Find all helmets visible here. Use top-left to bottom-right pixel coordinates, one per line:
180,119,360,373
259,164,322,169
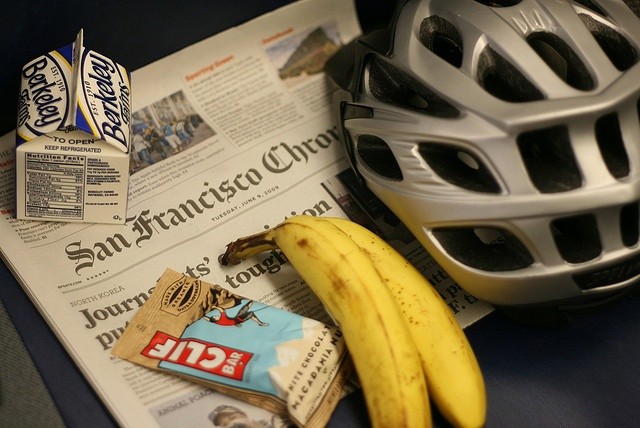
340,0,639,314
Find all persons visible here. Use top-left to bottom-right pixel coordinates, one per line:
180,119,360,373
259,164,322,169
129,113,194,176
208,405,270,428
202,300,269,327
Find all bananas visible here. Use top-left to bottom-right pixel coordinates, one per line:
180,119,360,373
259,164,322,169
223,215,488,428
